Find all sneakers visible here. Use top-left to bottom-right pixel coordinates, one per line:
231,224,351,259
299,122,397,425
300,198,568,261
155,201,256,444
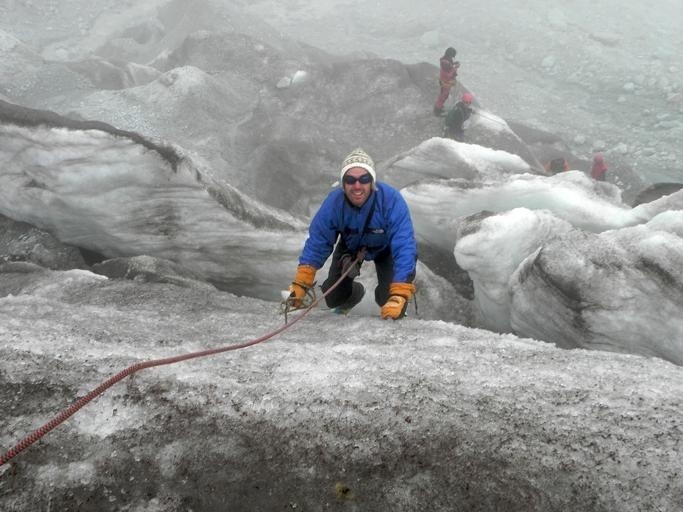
334,282,364,315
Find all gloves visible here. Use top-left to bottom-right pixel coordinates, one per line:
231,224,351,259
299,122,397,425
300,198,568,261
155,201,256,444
289,264,315,308
382,283,415,320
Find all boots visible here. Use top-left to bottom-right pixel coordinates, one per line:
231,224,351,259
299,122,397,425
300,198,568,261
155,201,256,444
434,107,446,117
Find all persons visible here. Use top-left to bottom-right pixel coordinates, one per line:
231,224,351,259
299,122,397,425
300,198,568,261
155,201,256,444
545,159,569,176
434,48,460,118
289,149,419,321
444,92,476,141
593,154,608,179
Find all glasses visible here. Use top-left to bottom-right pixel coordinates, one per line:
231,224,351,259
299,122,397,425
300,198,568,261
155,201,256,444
343,174,371,184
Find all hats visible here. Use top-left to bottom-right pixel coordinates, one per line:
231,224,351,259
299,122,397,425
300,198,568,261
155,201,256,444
445,47,456,57
341,147,376,191
462,94,471,103
